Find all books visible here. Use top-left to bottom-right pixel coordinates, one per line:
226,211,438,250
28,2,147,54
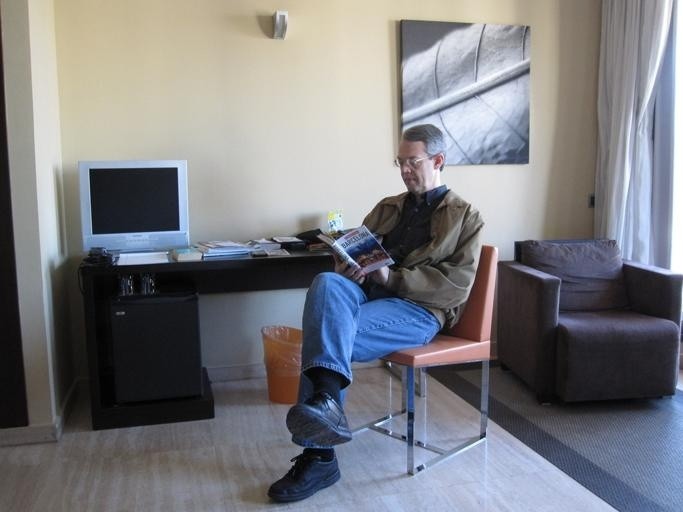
172,238,281,263
316,225,395,278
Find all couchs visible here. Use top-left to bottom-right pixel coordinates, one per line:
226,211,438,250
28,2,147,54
497,238,682,405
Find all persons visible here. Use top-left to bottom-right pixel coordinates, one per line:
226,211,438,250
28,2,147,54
265,124,485,503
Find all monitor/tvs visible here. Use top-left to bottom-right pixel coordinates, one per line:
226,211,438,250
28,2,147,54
79,160,189,252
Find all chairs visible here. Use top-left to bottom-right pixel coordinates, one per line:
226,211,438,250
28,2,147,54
349,238,499,475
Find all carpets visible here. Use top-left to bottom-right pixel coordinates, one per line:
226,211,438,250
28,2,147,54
423,358,681,510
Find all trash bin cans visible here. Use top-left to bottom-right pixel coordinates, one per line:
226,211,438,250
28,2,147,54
261,325,304,404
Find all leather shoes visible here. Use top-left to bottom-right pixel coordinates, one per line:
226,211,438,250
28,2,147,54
286,392,352,445
266,449,341,502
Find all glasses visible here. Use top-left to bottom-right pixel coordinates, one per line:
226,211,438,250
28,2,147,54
394,155,434,169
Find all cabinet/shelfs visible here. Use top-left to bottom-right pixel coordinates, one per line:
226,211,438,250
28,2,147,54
78,239,350,430
